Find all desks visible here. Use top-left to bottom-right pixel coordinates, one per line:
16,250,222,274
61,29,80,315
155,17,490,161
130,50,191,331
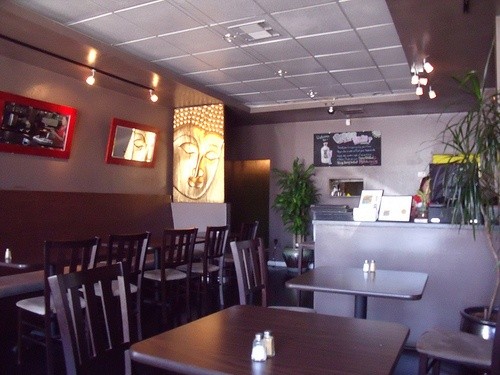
0,241,204,298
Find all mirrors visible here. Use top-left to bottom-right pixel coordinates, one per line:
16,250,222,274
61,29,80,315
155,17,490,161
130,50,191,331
104,118,161,169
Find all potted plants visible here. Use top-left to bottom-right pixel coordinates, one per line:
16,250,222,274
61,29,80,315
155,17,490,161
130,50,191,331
417,191,430,219
434,70,500,340
270,157,322,276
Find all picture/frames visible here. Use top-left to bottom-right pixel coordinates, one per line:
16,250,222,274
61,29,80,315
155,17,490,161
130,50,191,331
1,90,78,159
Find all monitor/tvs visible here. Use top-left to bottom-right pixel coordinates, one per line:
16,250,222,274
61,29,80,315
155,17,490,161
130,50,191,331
429,163,478,200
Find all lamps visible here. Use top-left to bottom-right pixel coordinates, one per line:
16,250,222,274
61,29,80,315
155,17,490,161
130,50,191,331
149,89,158,103
86,70,96,86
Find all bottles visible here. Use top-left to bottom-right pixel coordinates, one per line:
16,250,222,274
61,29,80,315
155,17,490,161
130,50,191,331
363,272,368,280
251,362,265,375
370,260,376,272
321,143,330,164
363,260,369,272
5,248,11,258
5,259,11,263
370,272,375,280
251,333,266,361
263,330,275,357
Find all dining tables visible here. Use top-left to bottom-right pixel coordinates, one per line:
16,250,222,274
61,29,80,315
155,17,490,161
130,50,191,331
286,263,429,319
124,304,411,375
1,261,33,273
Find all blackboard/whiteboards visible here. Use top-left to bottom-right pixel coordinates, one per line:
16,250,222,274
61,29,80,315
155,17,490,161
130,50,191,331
312,131,382,167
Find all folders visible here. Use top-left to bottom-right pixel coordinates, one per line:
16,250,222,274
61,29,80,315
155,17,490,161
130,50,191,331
310,203,354,221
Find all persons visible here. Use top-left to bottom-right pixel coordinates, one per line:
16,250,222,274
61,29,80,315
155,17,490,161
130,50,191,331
412,176,429,217
1,102,68,151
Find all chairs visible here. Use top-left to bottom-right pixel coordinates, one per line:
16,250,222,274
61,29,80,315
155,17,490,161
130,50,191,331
16,237,102,365
144,221,259,331
230,237,268,306
95,231,151,347
47,259,135,375
416,284,500,374
